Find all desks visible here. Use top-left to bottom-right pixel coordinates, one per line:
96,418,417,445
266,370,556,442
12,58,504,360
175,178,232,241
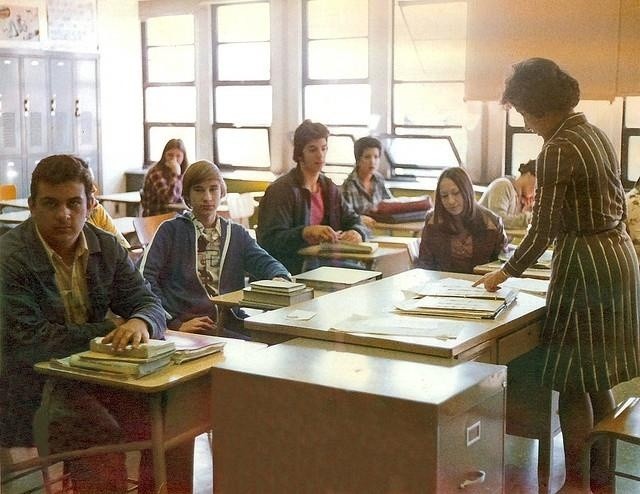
0,198,28,214
31,330,268,494
473,259,552,280
298,242,413,280
372,221,425,239
244,268,562,494
163,202,251,229
209,276,338,346
111,216,142,250
0,210,31,223
504,229,527,239
96,190,143,204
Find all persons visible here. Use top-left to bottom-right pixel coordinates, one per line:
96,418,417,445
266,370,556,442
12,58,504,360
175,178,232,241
0,154,194,494
139,139,190,217
477,158,537,231
625,177,640,262
137,161,294,341
256,120,369,274
78,157,133,253
472,58,640,494
417,168,508,273
340,136,397,229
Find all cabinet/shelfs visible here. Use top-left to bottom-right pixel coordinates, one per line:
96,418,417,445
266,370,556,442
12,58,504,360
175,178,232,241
0,48,104,212
211,337,508,494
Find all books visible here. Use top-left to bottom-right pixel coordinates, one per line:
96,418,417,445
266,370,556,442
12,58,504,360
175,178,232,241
319,239,379,254
239,279,314,308
296,265,382,292
390,277,519,319
498,243,554,269
50,328,226,380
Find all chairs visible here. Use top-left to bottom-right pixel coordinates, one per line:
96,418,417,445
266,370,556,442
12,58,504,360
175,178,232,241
581,396,640,494
0,183,18,204
133,212,180,250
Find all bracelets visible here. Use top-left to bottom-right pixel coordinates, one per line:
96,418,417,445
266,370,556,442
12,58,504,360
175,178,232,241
501,268,509,279
